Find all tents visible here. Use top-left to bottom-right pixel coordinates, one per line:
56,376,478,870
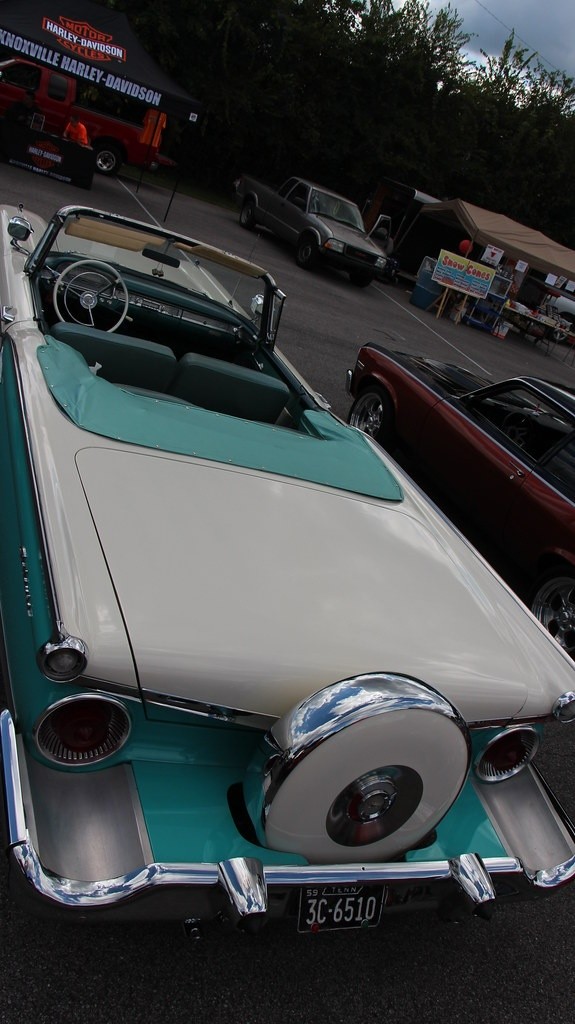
0,0,207,221
393,198,575,331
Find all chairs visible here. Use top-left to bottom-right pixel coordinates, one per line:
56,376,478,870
54,324,178,392
172,350,291,425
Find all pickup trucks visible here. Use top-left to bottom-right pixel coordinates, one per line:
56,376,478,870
237,173,392,289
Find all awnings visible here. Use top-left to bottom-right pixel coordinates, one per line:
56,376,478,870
386,178,444,244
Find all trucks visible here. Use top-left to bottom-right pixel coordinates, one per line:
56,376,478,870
0,58,178,178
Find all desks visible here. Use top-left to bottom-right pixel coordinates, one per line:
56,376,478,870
491,303,575,369
0,119,94,191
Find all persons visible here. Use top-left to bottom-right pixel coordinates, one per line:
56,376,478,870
64,114,89,146
5,90,43,130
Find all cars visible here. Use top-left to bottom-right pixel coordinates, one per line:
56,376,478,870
1,202,575,932
347,339,575,664
540,293,575,345
